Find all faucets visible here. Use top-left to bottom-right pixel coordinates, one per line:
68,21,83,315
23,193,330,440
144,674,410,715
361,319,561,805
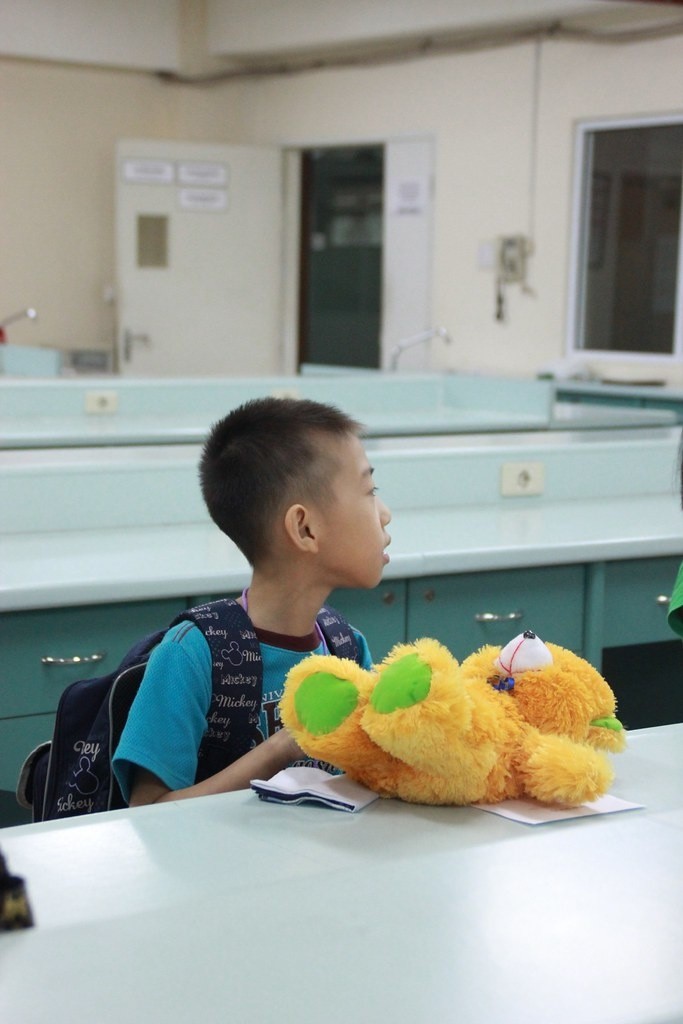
0,307,39,327
379,326,452,373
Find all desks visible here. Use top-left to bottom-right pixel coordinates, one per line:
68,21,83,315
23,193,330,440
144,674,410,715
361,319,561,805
0,441,683,612
0,374,558,453
0,722,682,1024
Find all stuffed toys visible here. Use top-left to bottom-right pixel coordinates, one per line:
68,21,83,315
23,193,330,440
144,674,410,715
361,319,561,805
276,628,628,811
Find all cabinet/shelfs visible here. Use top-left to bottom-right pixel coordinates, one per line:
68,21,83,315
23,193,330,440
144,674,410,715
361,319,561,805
214,561,588,762
588,550,683,675
0,598,190,828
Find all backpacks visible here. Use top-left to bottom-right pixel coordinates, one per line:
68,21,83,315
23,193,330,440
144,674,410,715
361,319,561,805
17,599,360,822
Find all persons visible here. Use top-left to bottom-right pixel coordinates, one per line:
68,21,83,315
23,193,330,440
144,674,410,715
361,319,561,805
110,396,394,810
